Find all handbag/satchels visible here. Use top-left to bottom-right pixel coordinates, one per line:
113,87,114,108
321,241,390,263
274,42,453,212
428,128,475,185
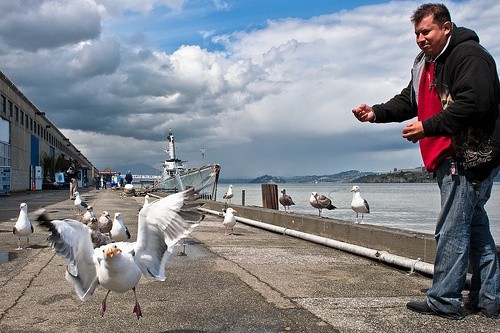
456,131,500,181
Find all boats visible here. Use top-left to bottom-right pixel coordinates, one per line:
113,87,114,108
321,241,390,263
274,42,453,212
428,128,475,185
132,127,221,202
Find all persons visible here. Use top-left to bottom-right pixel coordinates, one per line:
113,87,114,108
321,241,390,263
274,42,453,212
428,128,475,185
67,161,80,199
352,3,500,319
102,174,107,189
117,171,124,189
126,170,133,184
95,173,100,189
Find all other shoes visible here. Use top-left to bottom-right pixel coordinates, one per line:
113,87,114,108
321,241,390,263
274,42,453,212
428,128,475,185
464,301,500,319
407,300,436,314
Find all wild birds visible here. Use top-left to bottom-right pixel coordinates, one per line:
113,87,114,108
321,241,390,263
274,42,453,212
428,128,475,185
223,183,233,203
112,211,131,242
287,211,287,212
351,185,369,224
74,191,87,213
310,191,337,216
279,188,295,212
82,205,94,224
13,202,34,249
87,216,99,231
98,210,112,235
223,207,237,230
36,187,204,320
